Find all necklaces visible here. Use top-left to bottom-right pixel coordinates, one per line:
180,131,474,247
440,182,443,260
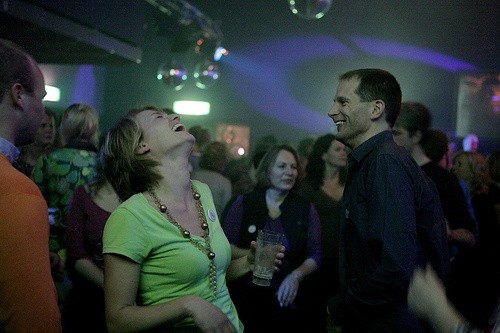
148,180,218,298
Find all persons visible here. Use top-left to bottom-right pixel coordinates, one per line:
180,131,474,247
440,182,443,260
100,105,244,333
326,69,446,333
408,264,500,333
0,43,63,333
223,144,321,333
186,102,500,246
18,103,130,333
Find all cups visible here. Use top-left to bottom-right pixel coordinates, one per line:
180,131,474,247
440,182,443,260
252,230,282,288
47,207,61,227
